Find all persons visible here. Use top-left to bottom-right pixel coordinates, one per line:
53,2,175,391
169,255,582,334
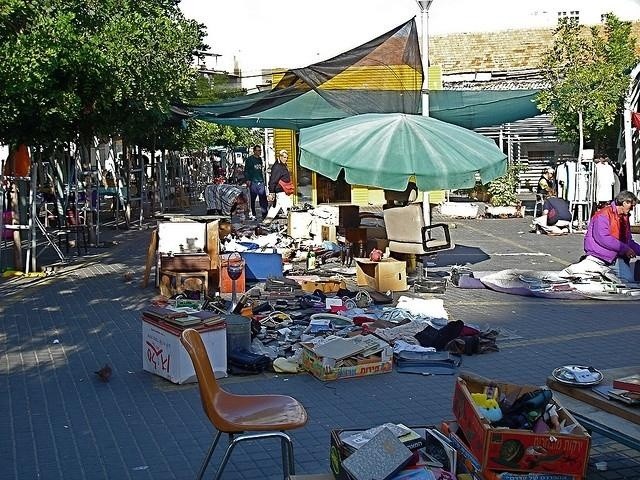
578,190,640,286
243,144,268,219
202,184,248,224
537,166,556,220
538,192,572,228
261,148,295,224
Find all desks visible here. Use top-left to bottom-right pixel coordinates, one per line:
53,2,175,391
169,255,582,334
220,247,283,294
545,358,640,454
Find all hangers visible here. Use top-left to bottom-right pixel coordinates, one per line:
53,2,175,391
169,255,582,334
597,152,611,165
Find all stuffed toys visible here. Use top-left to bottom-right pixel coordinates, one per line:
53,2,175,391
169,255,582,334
471,389,505,422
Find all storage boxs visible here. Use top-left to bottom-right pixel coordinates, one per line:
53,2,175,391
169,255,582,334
139,303,231,385
284,204,409,293
329,420,569,480
452,373,592,480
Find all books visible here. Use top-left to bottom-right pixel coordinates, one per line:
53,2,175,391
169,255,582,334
309,332,385,364
332,420,472,480
139,304,227,332
589,371,640,406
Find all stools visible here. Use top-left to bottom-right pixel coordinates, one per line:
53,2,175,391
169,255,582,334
156,267,210,297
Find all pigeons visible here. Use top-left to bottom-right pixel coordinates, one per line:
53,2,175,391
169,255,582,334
94,362,115,384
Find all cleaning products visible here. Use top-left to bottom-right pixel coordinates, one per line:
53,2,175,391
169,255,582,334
359,240,366,256
340,247,345,265
346,243,353,266
307,244,316,270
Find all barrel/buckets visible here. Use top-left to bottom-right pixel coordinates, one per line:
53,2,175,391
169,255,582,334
224,314,252,355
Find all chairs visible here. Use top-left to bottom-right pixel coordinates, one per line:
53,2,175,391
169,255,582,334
380,200,456,289
532,191,549,219
179,326,310,479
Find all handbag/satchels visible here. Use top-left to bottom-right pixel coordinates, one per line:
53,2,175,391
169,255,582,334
278,176,295,195
546,208,559,225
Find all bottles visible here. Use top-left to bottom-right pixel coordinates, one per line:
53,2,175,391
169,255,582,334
308,248,316,270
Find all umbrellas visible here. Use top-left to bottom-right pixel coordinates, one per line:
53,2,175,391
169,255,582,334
297,111,509,194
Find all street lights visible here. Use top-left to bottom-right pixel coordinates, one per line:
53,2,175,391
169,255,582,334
415,0,433,233
